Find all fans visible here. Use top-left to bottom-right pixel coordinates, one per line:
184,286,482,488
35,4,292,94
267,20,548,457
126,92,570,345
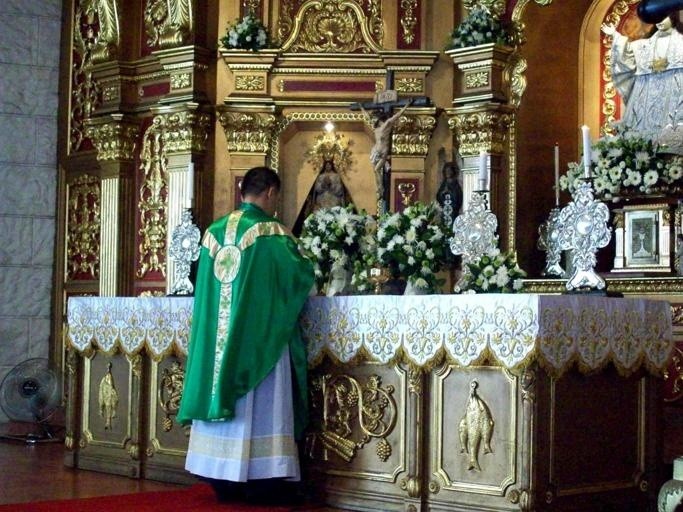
1,357,66,445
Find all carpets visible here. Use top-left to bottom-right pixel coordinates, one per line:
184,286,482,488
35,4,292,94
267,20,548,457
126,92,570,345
0,483,329,512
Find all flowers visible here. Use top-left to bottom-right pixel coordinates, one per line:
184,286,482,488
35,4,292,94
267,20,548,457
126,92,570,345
446,6,515,47
376,201,453,287
291,207,377,293
559,122,683,196
453,236,526,293
219,14,280,49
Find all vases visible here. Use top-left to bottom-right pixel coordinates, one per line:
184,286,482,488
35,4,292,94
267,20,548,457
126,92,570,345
403,277,433,293
329,269,355,295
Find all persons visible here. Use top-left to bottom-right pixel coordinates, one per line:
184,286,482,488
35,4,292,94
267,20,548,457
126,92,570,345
292,141,358,238
357,96,413,200
437,162,462,219
599,7,682,140
173,165,316,507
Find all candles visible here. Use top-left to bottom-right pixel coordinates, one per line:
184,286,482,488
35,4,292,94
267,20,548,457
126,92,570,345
554,142,560,197
188,163,194,200
582,125,590,178
480,150,487,181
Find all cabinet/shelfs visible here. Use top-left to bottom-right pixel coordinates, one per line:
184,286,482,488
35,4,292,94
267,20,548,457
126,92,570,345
63,294,663,512
